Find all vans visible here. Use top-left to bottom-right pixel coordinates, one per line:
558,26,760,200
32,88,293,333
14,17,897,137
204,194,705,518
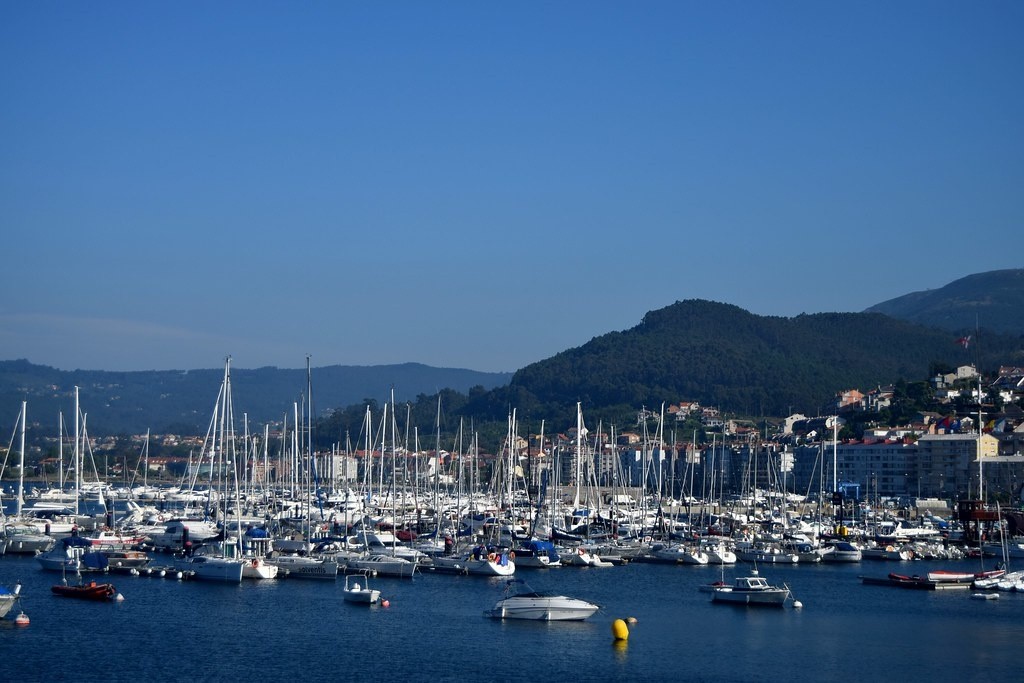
614,494,636,504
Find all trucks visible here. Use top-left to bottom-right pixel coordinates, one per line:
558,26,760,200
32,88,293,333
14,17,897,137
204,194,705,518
0,353,1024,589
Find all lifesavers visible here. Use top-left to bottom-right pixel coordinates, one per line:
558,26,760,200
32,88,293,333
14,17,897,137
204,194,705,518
645,536,651,541
665,520,670,525
510,552,515,558
322,524,328,531
578,548,585,556
252,560,259,568
158,516,163,521
488,553,495,560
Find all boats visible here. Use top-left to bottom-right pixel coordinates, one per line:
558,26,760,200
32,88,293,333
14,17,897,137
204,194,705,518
713,576,790,607
973,593,999,600
0,587,21,619
484,578,600,621
344,574,380,602
51,573,117,599
